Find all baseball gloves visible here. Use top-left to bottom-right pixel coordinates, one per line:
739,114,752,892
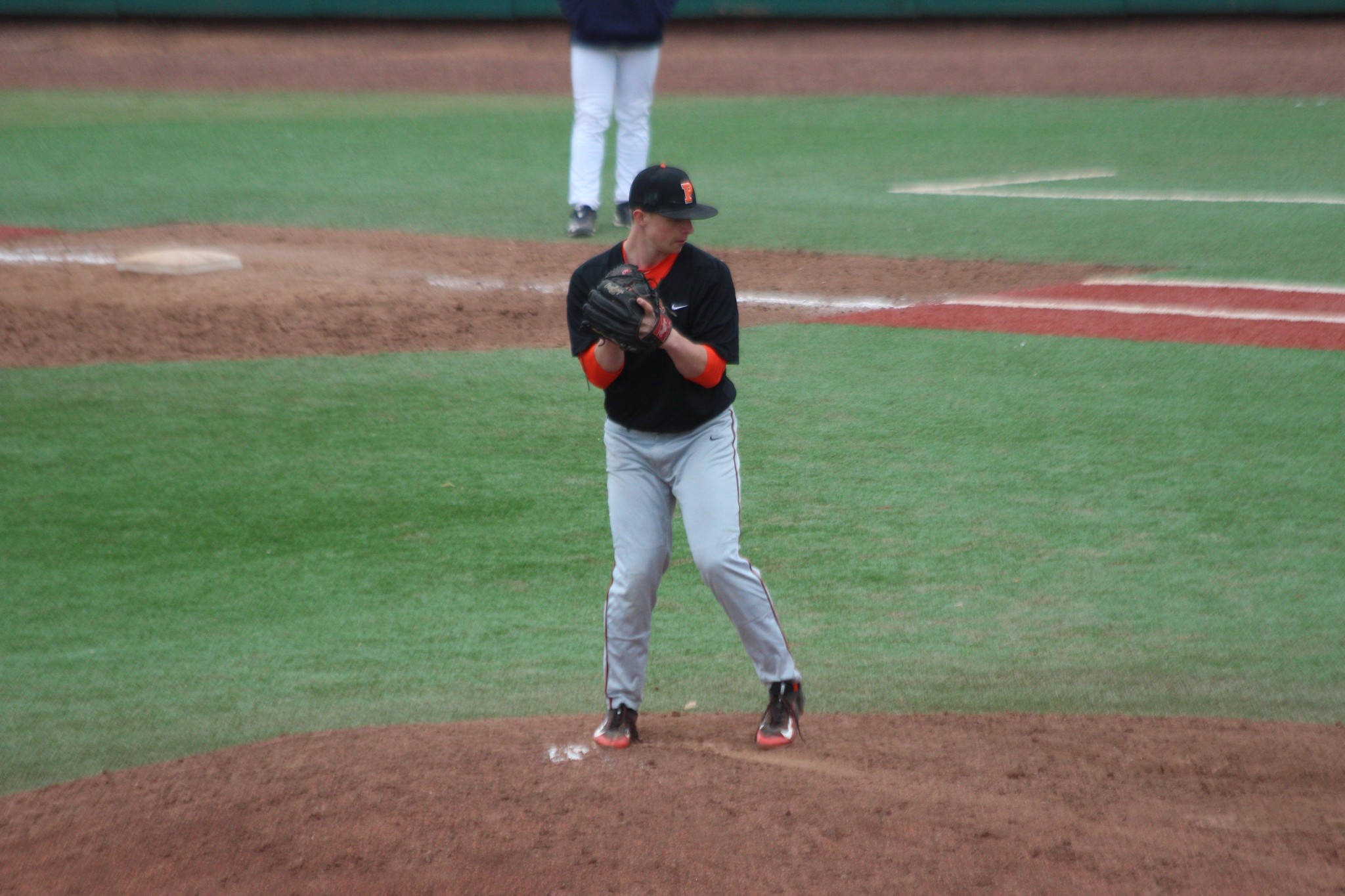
582,264,674,352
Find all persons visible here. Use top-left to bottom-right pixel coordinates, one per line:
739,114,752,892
554,0,676,239
566,163,806,747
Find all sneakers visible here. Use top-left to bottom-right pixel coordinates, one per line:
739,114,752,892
612,201,633,227
594,702,639,750
755,679,808,750
566,205,597,237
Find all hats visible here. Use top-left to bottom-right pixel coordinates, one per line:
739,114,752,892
629,163,719,220
